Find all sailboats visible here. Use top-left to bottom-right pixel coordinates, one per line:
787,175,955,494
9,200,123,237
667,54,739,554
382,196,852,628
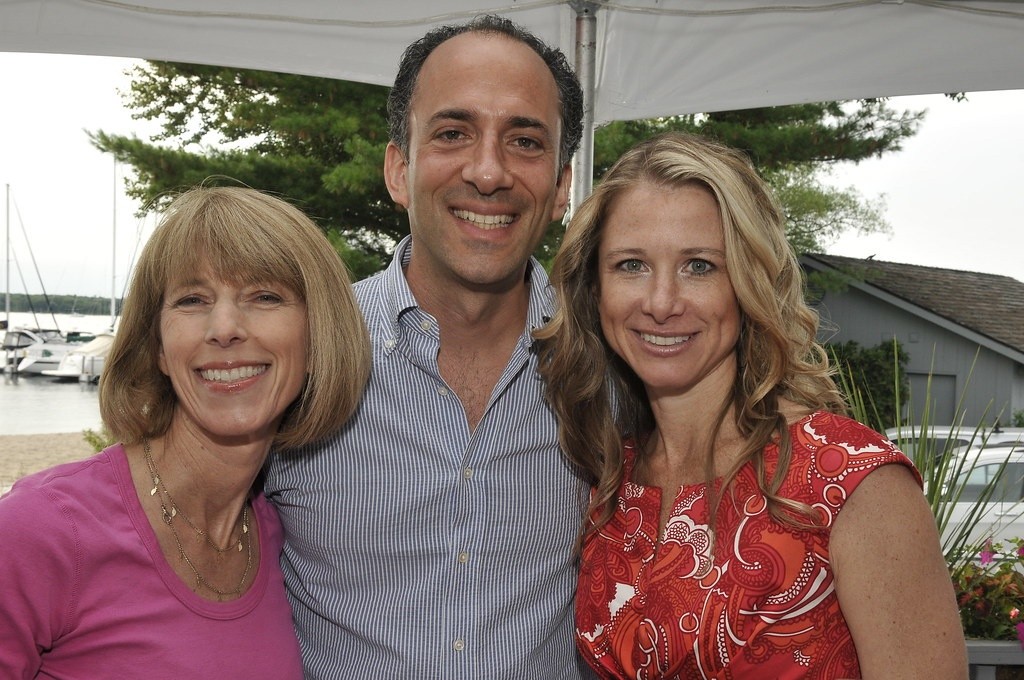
0,140,164,383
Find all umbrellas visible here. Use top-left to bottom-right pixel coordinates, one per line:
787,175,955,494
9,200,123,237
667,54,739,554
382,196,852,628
0,0,1024,224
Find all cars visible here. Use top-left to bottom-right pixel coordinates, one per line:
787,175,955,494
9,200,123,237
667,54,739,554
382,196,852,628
884,424,1024,585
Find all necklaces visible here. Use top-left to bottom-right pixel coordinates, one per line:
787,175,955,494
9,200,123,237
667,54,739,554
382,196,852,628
141,440,253,603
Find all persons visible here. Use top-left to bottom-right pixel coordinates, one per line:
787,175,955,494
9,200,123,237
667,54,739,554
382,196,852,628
532,131,967,680
263,18,615,680
0,185,370,680
